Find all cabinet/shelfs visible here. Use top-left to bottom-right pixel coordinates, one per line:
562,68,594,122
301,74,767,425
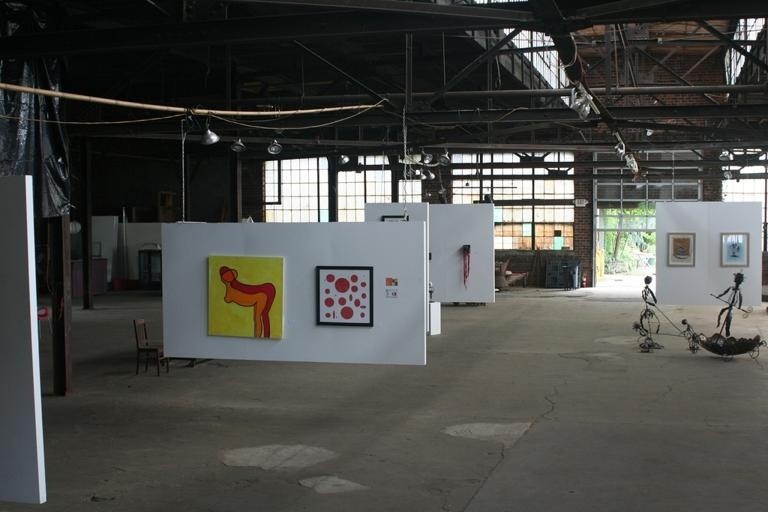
70,257,107,299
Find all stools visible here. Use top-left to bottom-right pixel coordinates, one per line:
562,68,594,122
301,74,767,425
38,307,52,339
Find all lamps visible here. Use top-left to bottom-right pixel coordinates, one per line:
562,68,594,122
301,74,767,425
417,148,450,181
199,119,283,155
338,153,350,165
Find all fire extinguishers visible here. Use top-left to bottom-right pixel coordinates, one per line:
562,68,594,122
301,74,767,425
581,271,587,287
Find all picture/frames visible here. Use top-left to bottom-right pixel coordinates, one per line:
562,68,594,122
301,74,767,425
315,265,375,328
666,233,696,268
720,232,750,267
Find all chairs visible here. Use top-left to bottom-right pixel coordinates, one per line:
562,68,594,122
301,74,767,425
133,317,169,377
